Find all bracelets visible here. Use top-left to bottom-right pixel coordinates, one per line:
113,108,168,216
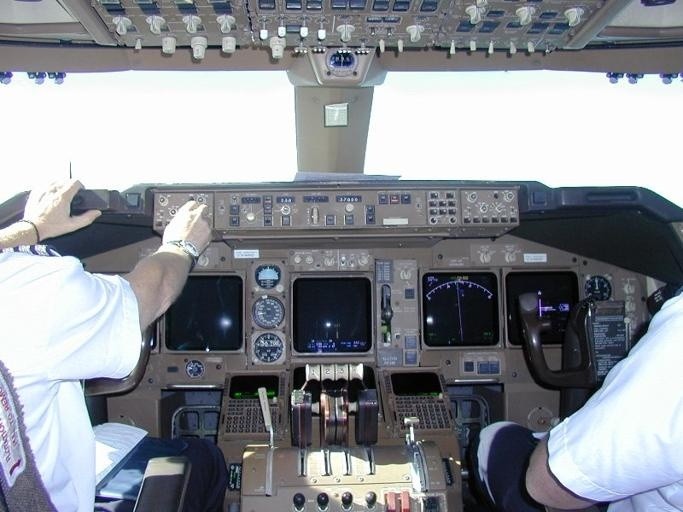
19,218,40,244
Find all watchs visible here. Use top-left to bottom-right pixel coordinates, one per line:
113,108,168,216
165,239,200,273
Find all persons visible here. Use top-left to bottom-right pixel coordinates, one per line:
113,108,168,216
0,176,230,512
467,289,683,512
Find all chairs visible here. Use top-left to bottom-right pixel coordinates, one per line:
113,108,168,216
0,359,192,512
